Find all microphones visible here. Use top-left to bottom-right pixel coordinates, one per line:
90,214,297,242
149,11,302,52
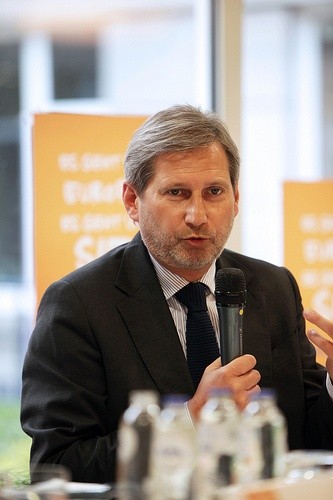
215,268,247,368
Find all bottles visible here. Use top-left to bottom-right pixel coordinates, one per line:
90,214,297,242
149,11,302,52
114,389,161,500
147,393,194,500
191,387,247,500
243,387,288,480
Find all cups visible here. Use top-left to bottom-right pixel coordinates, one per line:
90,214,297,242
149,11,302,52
0,466,70,500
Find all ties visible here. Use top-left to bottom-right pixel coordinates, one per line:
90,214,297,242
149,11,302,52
172,282,220,394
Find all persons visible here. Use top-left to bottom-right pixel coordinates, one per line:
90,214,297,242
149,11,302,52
21,104,333,481
